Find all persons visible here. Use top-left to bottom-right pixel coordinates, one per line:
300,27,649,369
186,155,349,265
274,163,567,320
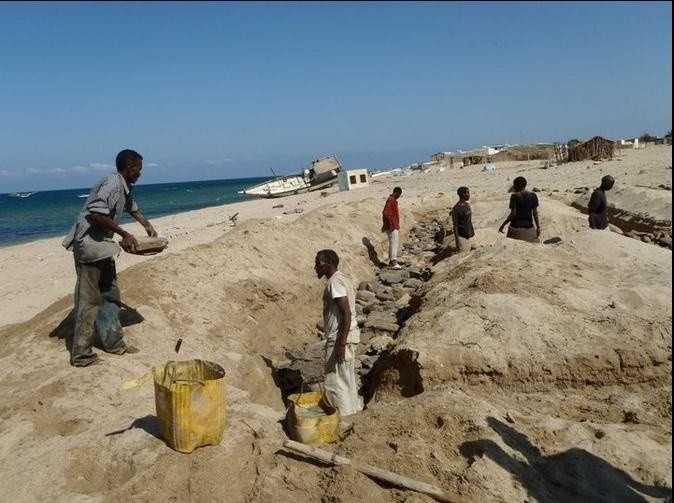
59,149,156,365
313,249,364,417
450,186,478,253
588,174,615,232
381,186,402,269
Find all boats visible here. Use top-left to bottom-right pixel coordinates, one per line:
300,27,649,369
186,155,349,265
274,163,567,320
8,190,40,198
237,153,342,198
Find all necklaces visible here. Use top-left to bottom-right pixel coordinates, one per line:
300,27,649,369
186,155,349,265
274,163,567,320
499,176,541,243
